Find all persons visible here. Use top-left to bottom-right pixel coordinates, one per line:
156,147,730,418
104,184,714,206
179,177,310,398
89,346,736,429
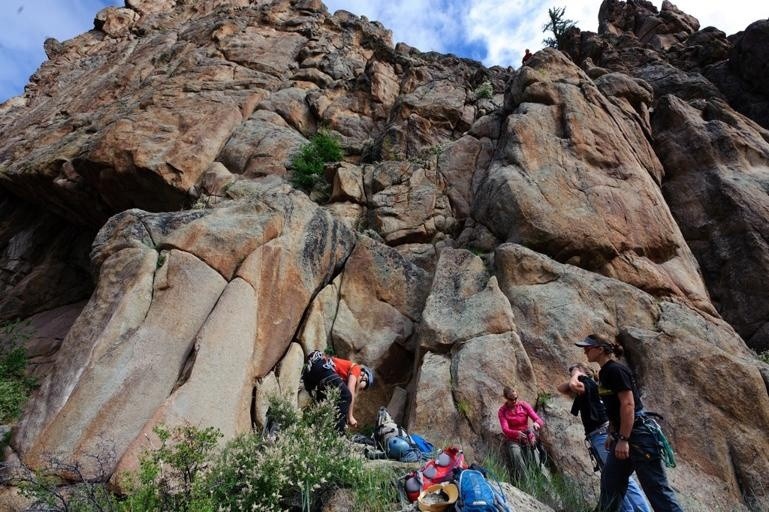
574,332,686,511
302,349,374,435
498,385,565,511
558,360,652,511
522,48,533,65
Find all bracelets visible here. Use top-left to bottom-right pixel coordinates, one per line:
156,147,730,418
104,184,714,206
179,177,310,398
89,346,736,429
617,432,629,441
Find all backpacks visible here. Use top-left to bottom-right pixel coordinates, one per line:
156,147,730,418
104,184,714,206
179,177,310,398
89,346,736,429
453,463,511,512
397,447,468,506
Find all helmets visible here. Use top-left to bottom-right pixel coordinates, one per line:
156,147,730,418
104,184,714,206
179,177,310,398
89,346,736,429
360,367,374,391
387,438,410,458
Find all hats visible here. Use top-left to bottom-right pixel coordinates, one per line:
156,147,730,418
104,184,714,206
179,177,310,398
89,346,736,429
415,482,459,512
574,336,601,349
